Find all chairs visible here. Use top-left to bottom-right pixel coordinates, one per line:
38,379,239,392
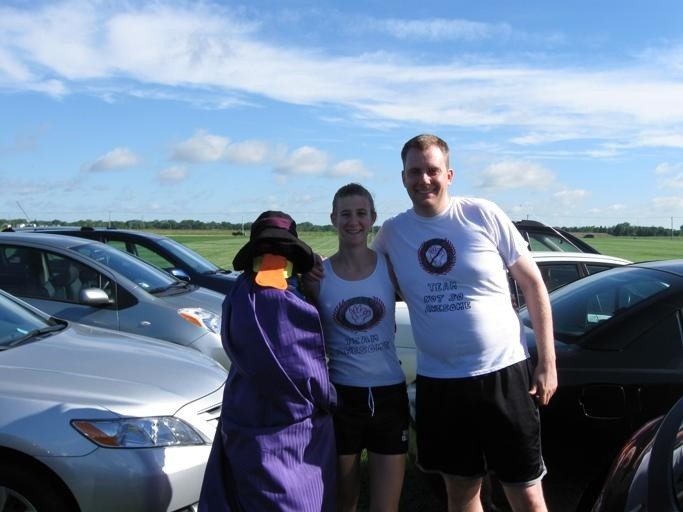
48,254,98,303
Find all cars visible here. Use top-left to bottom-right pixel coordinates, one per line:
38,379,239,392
1,289,231,511
1,231,232,379
412,259,682,479
7,227,243,297
391,251,645,400
517,219,599,251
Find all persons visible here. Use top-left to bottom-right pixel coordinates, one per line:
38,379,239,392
192,210,342,512
297,181,521,511
293,131,560,511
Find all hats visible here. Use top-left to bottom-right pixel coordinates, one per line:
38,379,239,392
232,211,315,273
